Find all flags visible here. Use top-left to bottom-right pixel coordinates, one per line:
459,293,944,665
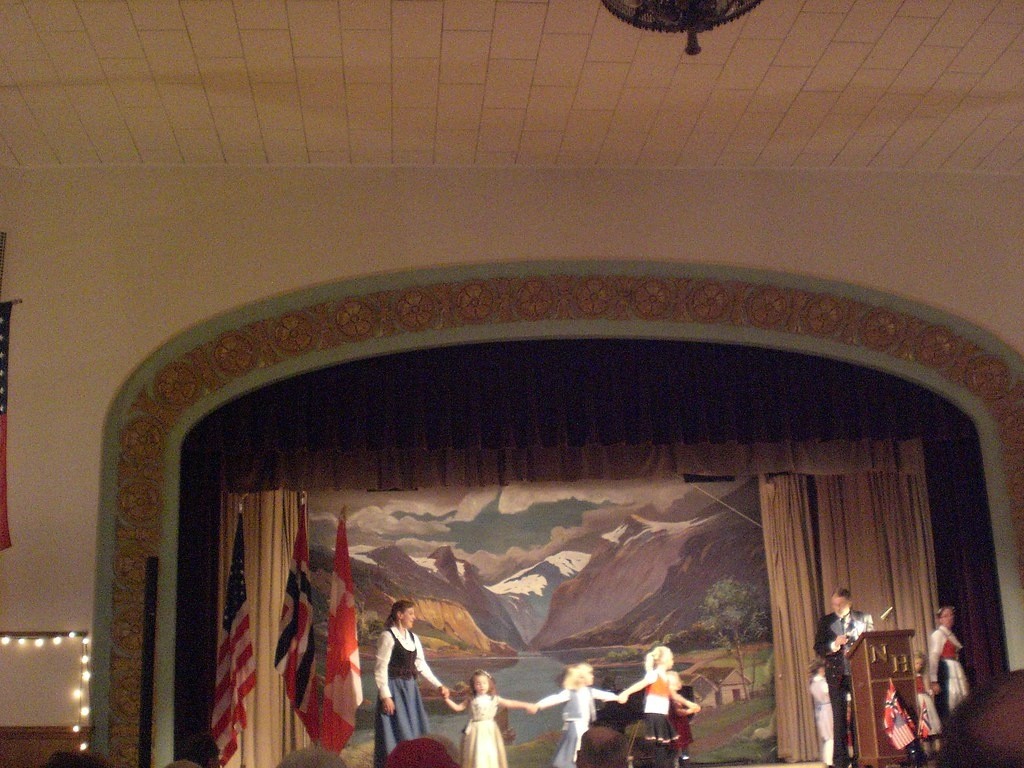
884,678,932,750
212,490,363,768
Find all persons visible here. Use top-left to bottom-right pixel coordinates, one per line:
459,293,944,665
374,600,701,768
937,667,1024,768
914,653,943,760
164,760,203,768
44,752,114,768
276,747,348,768
928,605,971,721
810,587,873,768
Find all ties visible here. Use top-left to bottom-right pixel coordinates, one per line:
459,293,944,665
840,618,847,635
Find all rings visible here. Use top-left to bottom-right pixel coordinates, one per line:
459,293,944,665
384,707,387,709
